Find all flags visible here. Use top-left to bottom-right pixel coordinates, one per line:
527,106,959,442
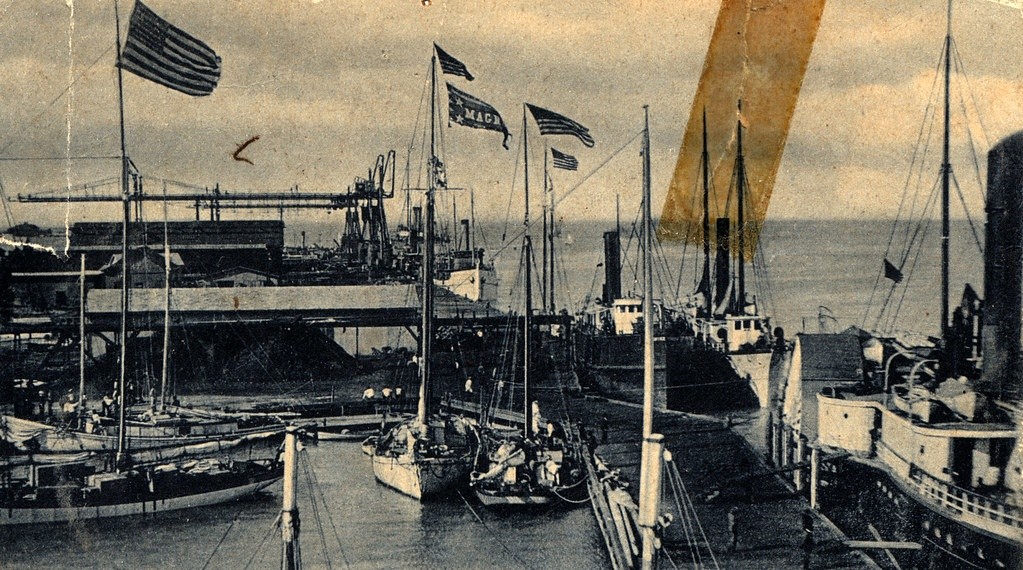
446,85,512,150
885,259,903,284
435,42,475,82
116,0,221,97
550,147,579,171
526,103,595,148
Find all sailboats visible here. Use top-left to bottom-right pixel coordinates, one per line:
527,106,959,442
577,96,770,411
819,2,1019,569
371,42,482,500
0,0,301,521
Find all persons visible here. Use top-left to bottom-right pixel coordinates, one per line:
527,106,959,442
40,376,181,438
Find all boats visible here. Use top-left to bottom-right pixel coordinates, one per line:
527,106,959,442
480,100,587,511
